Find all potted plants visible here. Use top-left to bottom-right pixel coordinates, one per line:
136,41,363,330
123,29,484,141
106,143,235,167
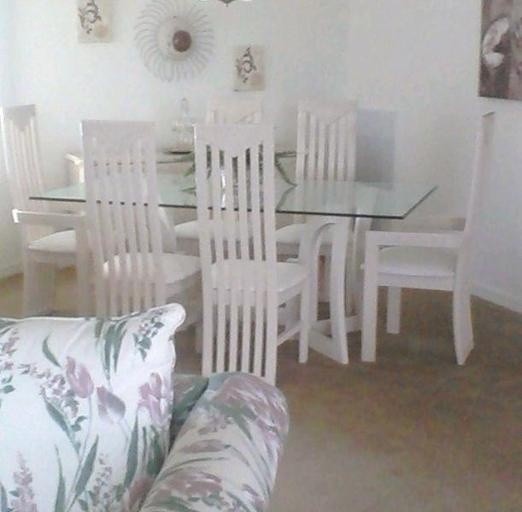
156,112,300,193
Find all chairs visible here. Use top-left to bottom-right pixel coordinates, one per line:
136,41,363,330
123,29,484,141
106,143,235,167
192,121,311,388
172,91,270,258
275,100,362,314
357,111,498,365
80,118,203,353
0,102,89,315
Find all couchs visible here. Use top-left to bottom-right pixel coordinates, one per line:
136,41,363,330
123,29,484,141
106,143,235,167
140,370,291,512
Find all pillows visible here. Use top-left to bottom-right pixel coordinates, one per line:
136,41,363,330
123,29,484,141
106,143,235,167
1,301,187,511
